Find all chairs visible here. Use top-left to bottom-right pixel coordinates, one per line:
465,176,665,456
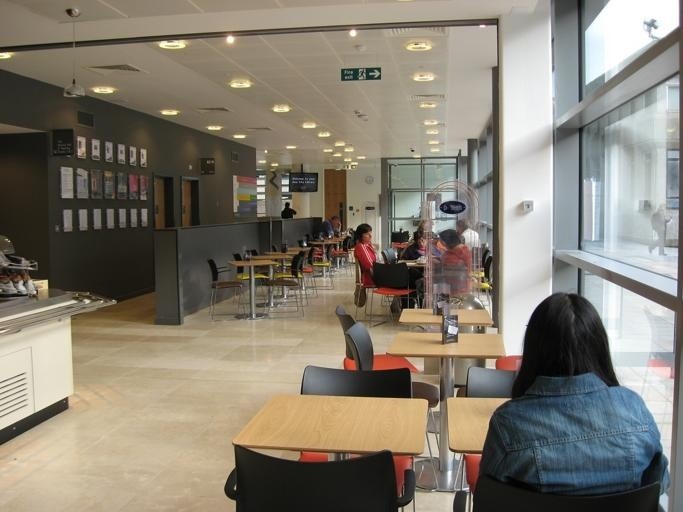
452,453,668,511
295,364,415,512
495,357,521,372
345,322,443,493
223,439,416,511
355,228,494,327
454,366,522,511
335,307,421,374
206,228,357,322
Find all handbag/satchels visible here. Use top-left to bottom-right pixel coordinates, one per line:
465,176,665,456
354,285,366,306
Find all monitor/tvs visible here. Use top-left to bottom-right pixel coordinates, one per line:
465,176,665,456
289,172,318,192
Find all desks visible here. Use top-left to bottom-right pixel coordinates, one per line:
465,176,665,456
387,331,505,492
445,395,514,455
399,307,495,433
232,391,430,465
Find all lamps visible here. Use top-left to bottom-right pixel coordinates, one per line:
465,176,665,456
63,9,85,97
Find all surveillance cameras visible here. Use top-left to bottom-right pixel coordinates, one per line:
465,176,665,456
409,145,417,153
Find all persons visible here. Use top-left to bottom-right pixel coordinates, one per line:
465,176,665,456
647,202,673,257
355,218,481,315
281,202,296,218
479,292,669,496
315,216,342,263
